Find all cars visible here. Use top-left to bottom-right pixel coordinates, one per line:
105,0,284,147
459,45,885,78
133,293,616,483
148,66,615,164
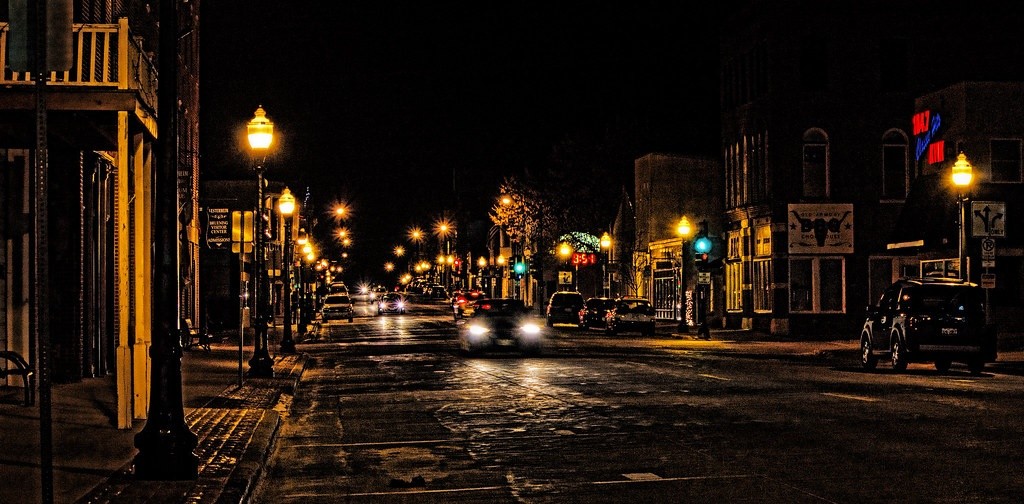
450,290,542,353
606,296,656,337
322,295,354,322
377,293,406,315
358,284,388,303
394,279,449,301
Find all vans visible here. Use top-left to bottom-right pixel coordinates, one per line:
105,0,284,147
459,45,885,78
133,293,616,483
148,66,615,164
329,283,348,295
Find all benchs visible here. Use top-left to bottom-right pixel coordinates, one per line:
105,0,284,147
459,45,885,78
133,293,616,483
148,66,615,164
178,318,212,352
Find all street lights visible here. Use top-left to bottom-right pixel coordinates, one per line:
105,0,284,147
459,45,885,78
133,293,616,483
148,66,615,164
950,155,974,276
601,233,611,297
561,241,569,291
297,233,322,333
278,187,299,355
248,104,276,376
499,254,505,299
480,257,486,291
678,216,692,331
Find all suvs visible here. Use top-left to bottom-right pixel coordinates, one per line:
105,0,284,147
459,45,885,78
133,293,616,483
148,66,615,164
860,278,997,374
544,290,585,327
579,298,616,332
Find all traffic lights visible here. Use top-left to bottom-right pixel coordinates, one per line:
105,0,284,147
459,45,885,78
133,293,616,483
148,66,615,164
702,254,709,271
454,260,459,271
